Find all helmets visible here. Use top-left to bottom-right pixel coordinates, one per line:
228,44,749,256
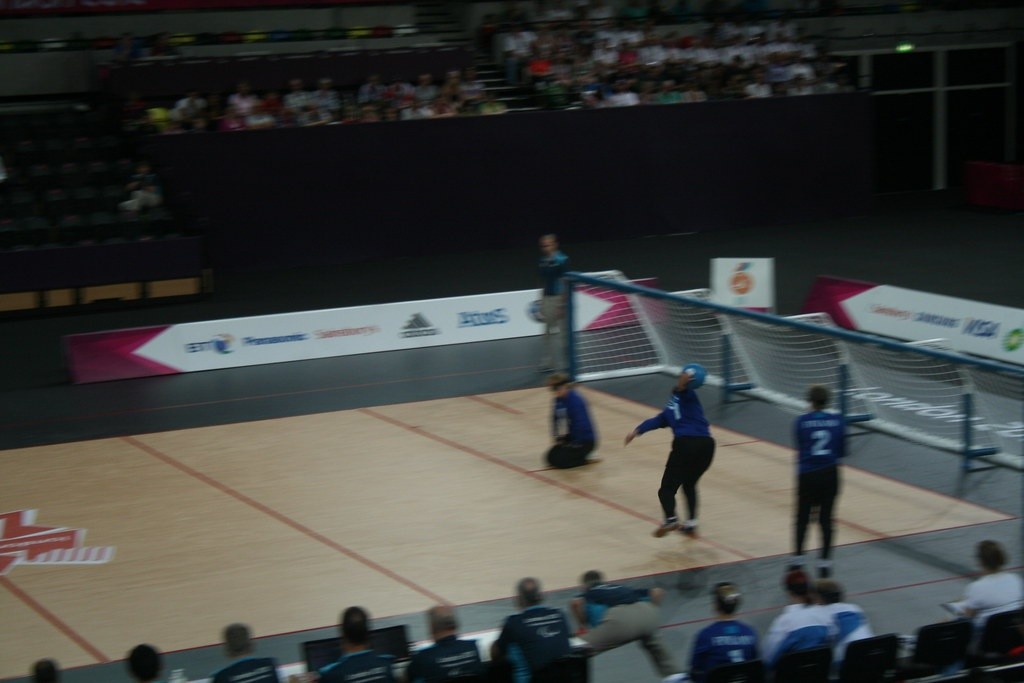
682,363,706,391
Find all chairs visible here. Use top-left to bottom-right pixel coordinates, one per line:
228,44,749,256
914,620,973,667
974,661,1024,683
977,610,1024,654
703,658,765,683
0,126,181,250
529,653,589,683
839,634,899,683
771,647,832,683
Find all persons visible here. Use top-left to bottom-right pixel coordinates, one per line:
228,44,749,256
307,606,402,683
402,604,486,683
945,539,1023,639
625,362,715,538
758,566,841,664
542,372,595,469
566,569,689,683
812,581,876,683
31,658,62,683
488,576,572,682
783,384,850,577
126,643,164,683
71,0,873,232
537,236,573,375
685,582,760,683
209,623,284,683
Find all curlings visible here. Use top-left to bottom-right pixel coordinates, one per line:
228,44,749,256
679,363,708,392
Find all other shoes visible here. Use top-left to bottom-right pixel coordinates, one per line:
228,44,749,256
789,555,805,571
680,518,699,533
817,558,831,579
653,518,679,537
